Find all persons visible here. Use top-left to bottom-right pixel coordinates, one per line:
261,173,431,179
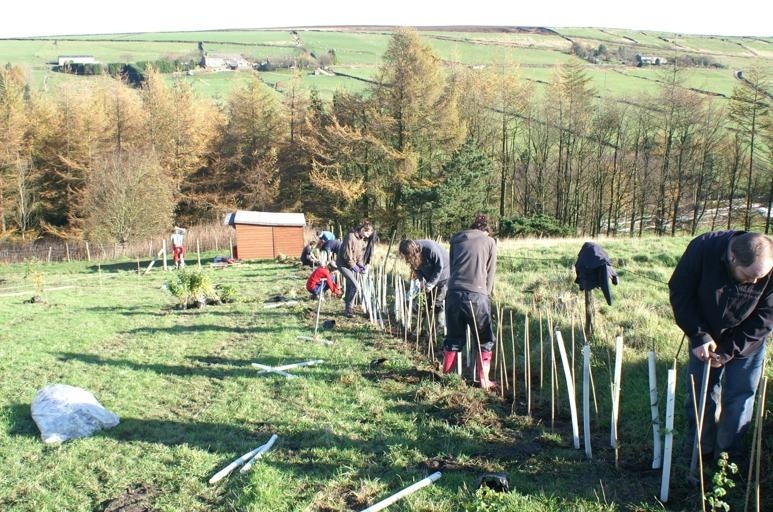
441,214,497,391
306,260,343,303
667,229,773,477
318,238,342,261
299,240,320,267
335,219,375,318
316,230,336,241
170,224,187,269
398,238,451,340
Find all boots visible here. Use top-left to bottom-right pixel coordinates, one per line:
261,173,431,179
476,350,497,390
343,299,356,318
442,349,457,375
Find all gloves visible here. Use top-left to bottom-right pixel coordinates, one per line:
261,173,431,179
356,263,366,273
351,265,359,272
